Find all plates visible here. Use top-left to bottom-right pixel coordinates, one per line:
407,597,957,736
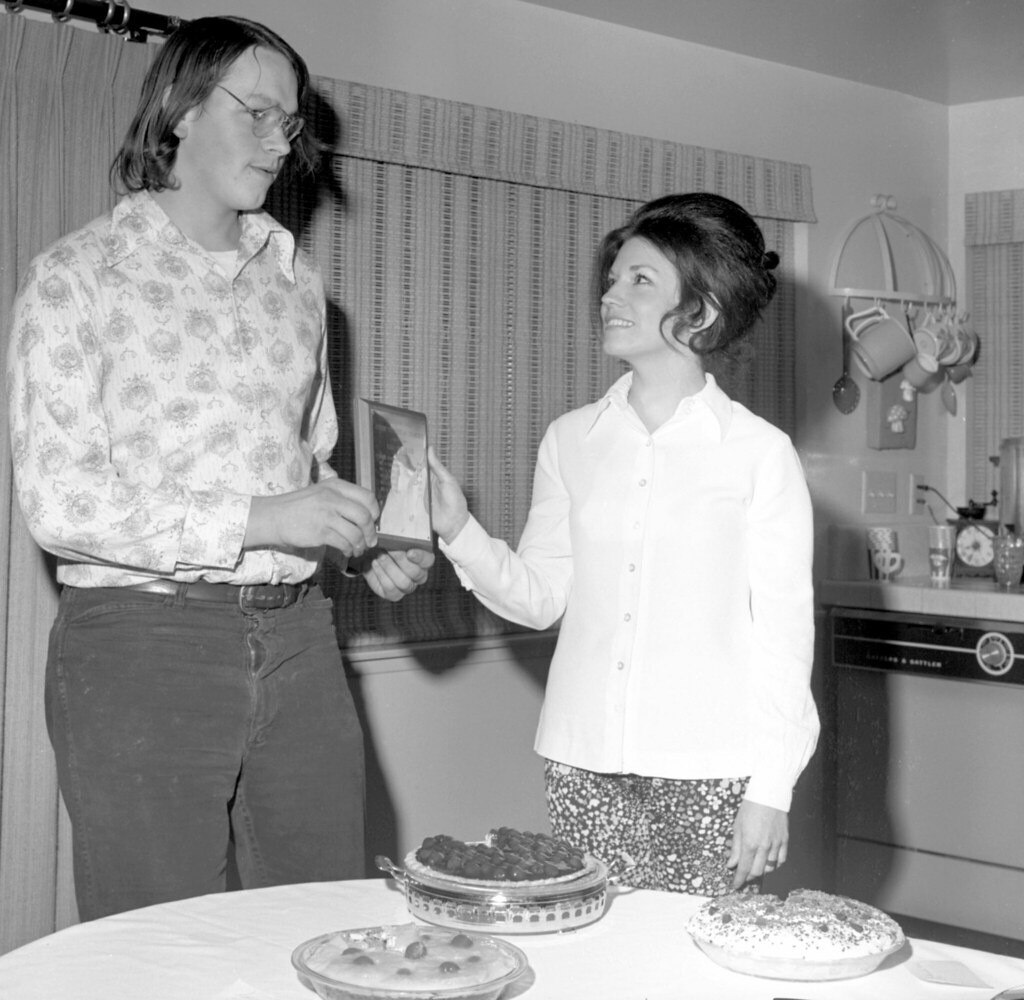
405,841,606,892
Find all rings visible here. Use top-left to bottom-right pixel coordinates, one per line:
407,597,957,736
766,859,777,867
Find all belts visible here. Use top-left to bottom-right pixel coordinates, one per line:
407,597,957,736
120,575,316,616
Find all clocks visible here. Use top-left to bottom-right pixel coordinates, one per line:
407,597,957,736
943,518,1010,582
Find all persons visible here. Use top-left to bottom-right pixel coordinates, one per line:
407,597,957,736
425,192,821,897
6,15,435,923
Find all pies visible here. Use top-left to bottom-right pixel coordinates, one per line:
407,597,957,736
305,920,516,999
404,827,595,887
688,887,896,978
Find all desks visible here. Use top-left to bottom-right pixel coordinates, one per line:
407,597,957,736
0,875,1024,1000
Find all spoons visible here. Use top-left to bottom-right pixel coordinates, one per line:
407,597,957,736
942,371,959,418
830,302,861,416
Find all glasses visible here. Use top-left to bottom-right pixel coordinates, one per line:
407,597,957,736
215,82,305,142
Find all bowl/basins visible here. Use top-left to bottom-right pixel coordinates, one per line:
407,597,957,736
689,900,906,982
292,925,527,999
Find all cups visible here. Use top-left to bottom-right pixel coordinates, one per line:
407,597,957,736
901,302,980,390
991,536,1024,588
925,525,959,583
865,526,901,582
843,306,915,381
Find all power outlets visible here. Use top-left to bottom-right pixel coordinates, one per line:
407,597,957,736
908,475,928,516
